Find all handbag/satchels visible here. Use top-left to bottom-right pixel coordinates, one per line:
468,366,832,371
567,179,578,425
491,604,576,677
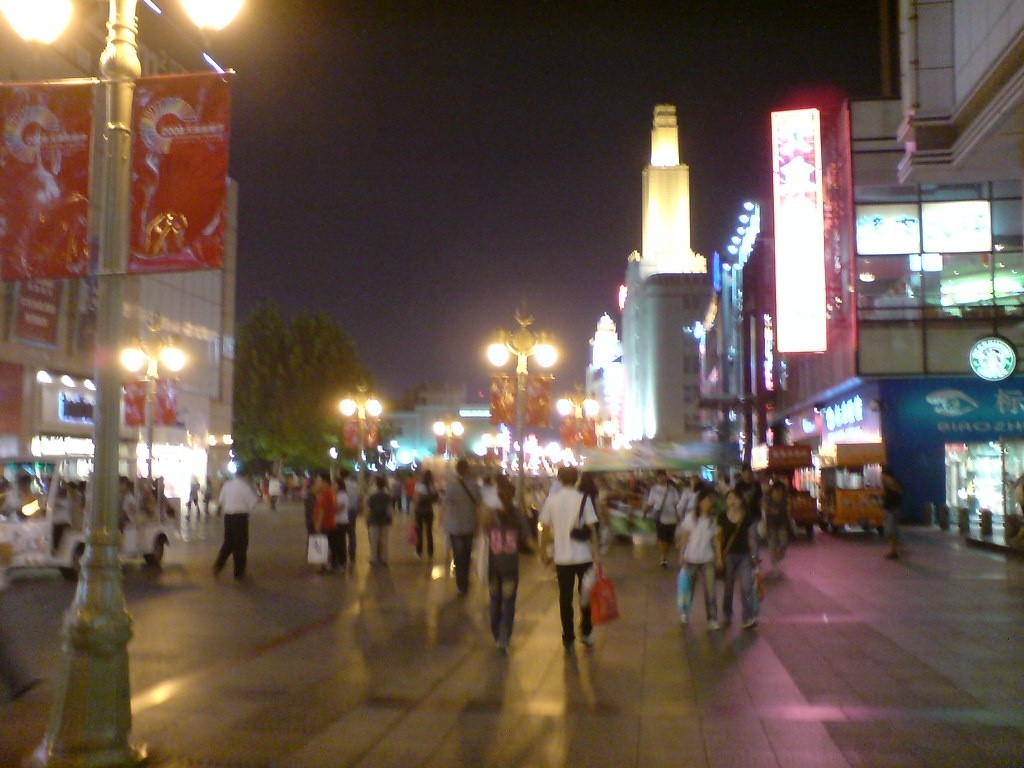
756,519,767,538
678,567,692,613
751,570,765,603
307,534,328,566
406,526,418,543
571,526,590,541
653,511,661,526
580,562,619,626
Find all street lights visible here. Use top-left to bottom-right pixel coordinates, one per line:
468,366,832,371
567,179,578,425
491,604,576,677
556,397,599,450
121,342,187,478
486,299,559,507
433,414,464,462
339,378,383,491
0,0,248,767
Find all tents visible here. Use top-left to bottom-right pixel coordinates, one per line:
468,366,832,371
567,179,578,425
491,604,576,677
579,441,741,472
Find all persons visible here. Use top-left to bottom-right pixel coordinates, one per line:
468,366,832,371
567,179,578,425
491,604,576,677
718,489,759,630
881,458,907,559
209,470,263,587
0,475,41,700
1010,474,1024,549
482,483,549,654
678,489,723,630
304,467,438,572
537,464,601,656
0,467,175,555
437,460,482,597
186,469,312,517
474,458,797,580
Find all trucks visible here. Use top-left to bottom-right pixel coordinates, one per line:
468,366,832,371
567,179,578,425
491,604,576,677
752,463,817,540
823,464,888,538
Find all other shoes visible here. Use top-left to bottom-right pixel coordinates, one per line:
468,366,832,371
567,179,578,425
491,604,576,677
707,619,721,631
741,618,758,628
496,641,510,655
561,639,576,650
722,613,731,625
659,559,668,568
576,635,593,647
680,614,689,623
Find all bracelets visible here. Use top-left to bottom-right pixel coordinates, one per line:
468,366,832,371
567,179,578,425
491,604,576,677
750,553,758,559
